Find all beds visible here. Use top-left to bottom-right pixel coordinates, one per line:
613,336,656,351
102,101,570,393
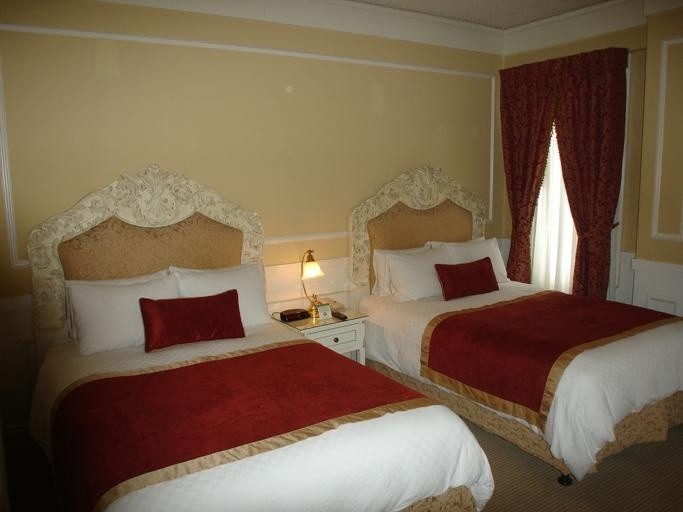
25,168,498,510
348,167,683,486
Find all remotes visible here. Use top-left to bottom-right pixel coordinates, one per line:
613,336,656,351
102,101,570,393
332,312,348,319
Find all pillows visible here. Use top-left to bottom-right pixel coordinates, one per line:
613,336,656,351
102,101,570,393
371,234,510,301
60,261,269,357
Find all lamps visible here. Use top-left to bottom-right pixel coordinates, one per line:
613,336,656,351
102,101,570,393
298,248,326,319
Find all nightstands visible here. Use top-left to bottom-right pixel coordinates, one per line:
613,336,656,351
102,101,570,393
278,307,369,369
